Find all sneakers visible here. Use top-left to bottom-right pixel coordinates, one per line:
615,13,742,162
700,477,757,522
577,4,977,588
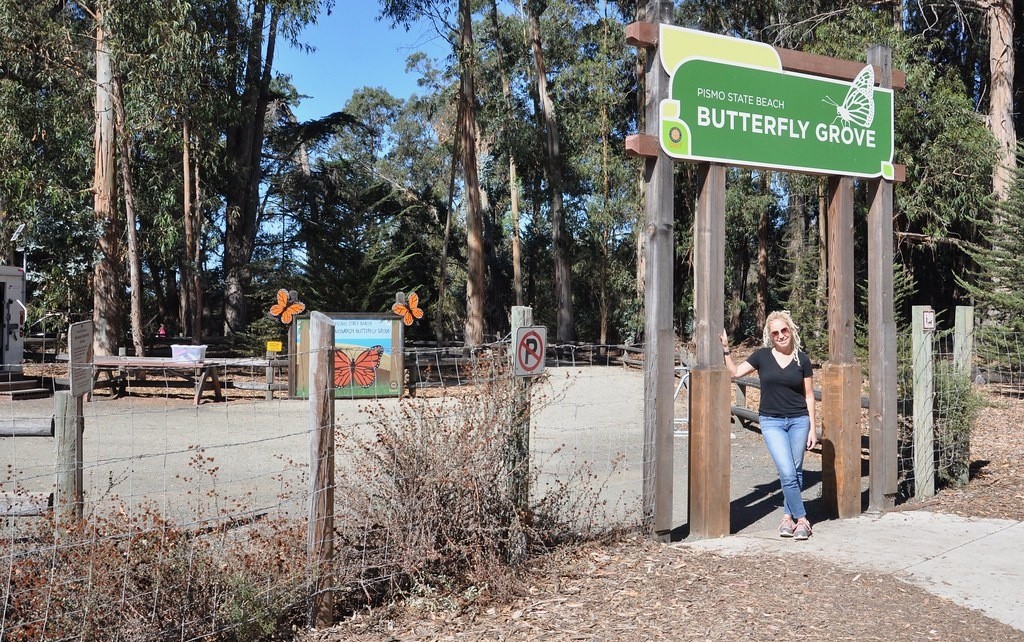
778,518,812,539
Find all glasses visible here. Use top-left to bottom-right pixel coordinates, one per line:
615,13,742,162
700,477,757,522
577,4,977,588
769,327,790,337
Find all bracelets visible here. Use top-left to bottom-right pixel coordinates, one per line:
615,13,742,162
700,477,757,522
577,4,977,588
724,350,731,355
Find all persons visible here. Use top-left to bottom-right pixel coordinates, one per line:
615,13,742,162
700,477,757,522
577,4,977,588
157,323,166,338
718,310,818,540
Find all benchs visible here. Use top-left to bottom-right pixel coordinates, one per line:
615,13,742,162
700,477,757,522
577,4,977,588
86,353,227,407
